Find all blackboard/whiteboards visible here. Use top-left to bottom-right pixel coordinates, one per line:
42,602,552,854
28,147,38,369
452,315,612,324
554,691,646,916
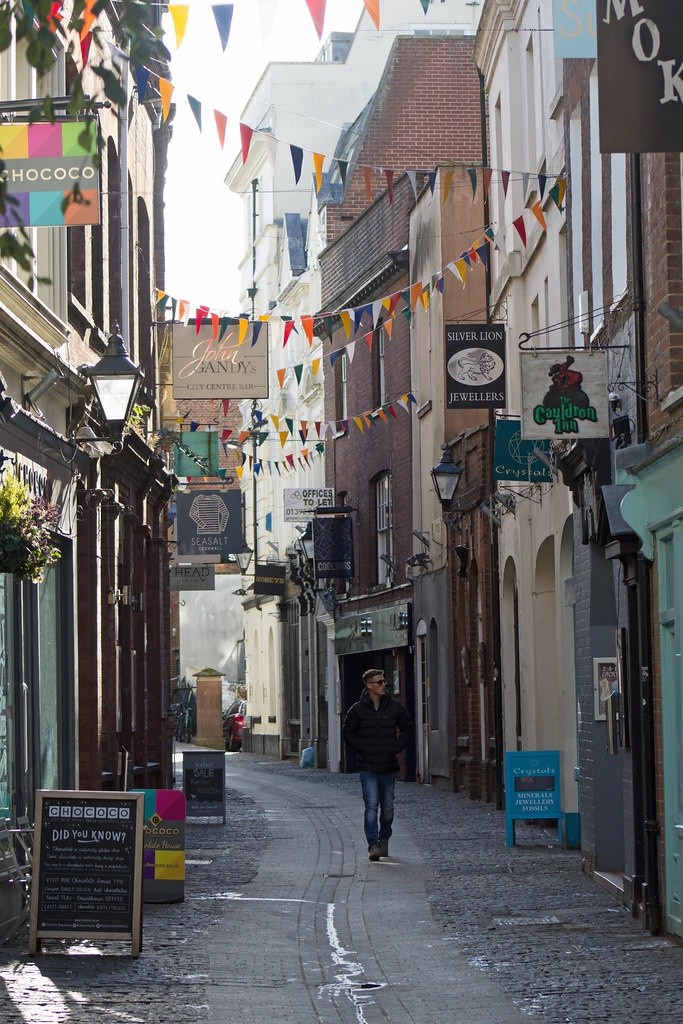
181,750,227,814
503,749,567,850
26,787,146,960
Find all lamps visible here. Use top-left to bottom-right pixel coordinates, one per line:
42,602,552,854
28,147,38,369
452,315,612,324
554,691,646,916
431,442,470,515
69,320,146,443
133,82,162,105
299,522,316,577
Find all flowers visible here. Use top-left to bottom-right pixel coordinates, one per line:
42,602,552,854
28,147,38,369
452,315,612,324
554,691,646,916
0,466,63,585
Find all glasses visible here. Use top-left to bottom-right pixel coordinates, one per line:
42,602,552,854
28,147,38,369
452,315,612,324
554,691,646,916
369,680,387,686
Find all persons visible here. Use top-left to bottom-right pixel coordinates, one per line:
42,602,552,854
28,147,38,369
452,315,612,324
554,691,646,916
341,669,415,861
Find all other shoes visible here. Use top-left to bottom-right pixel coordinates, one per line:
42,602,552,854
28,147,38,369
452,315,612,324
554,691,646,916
379,841,389,857
369,847,381,862
